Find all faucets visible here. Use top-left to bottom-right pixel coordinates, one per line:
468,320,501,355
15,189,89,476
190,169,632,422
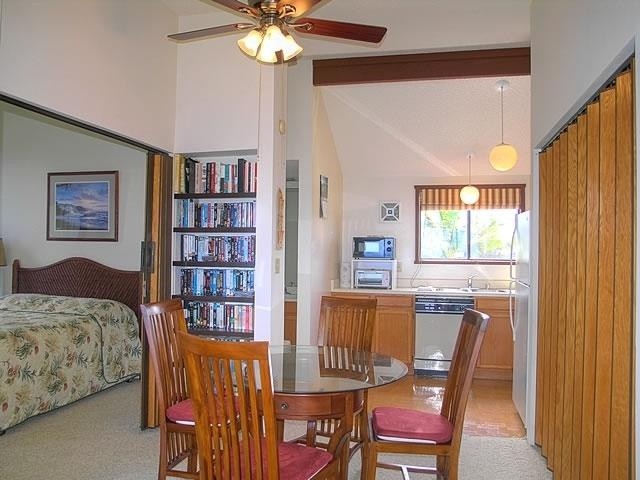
468,274,479,287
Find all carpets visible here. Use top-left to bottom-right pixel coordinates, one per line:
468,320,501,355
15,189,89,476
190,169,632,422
0,378,557,480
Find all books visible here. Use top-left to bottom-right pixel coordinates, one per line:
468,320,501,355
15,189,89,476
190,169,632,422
172,153,257,395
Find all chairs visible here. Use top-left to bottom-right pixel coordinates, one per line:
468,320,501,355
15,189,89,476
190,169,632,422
306,295,377,457
358,303,492,480
172,323,342,480
146,292,251,480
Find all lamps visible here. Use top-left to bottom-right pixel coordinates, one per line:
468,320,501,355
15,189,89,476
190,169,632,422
234,14,305,66
488,77,518,173
459,151,480,207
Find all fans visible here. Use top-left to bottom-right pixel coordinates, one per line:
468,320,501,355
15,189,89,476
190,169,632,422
160,1,388,51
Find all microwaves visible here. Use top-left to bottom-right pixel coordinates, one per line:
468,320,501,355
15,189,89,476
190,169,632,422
352,236,396,260
354,270,392,288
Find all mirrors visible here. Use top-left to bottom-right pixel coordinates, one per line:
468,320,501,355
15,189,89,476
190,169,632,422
283,157,300,350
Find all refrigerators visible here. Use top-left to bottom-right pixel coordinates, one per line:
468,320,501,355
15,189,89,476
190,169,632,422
506,211,532,429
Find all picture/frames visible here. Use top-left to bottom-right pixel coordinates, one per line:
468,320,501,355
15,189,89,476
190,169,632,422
45,171,121,242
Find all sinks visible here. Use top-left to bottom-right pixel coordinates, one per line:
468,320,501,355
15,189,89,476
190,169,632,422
435,288,469,292
472,289,512,293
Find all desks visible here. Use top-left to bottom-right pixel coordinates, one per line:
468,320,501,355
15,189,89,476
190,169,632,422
210,343,411,480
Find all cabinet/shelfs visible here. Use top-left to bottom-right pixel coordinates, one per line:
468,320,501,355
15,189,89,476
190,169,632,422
172,147,260,343
374,292,415,375
470,294,516,382
286,298,298,347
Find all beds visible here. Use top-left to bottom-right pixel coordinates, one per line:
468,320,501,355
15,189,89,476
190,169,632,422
2,257,145,436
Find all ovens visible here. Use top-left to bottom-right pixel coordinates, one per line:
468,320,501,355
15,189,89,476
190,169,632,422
412,298,475,372
